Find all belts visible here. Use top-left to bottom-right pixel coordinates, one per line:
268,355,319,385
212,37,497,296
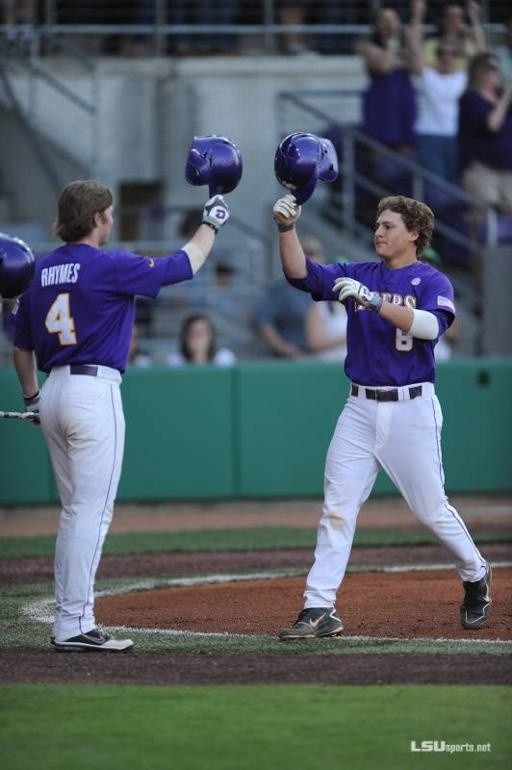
351,383,422,402
70,362,97,376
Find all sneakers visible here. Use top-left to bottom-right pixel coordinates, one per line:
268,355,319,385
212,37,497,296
461,560,493,630
56,629,135,652
278,606,344,640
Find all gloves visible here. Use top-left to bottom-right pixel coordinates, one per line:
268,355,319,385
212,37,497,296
272,194,301,233
201,193,230,234
22,390,41,424
332,276,381,310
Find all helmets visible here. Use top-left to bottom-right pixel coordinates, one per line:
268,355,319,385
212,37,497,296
185,134,242,198
0,234,36,299
274,132,339,204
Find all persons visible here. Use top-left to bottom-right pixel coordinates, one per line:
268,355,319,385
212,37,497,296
8,180,230,651
0,0,512,368
274,194,487,637
